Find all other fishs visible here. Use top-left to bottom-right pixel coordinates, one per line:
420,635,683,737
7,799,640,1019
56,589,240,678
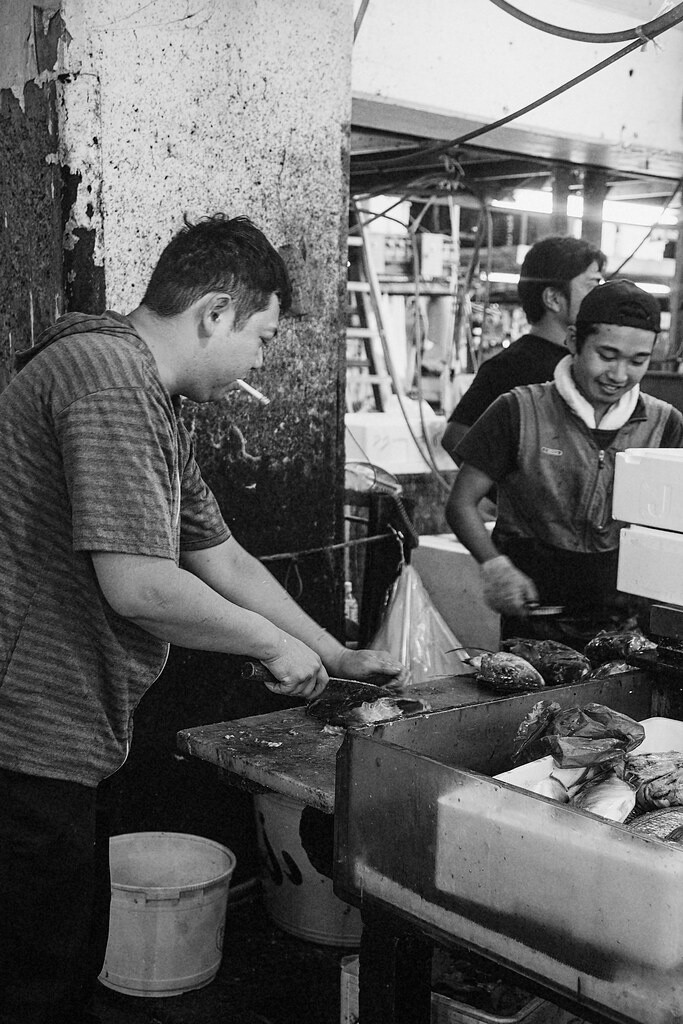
565,771,683,844
305,630,658,728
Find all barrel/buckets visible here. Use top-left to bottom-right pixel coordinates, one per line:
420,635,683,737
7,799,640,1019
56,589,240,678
98,831,236,997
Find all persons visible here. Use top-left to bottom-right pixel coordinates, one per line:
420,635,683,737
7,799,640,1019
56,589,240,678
0,214,412,1024
444,279,683,647
441,239,607,503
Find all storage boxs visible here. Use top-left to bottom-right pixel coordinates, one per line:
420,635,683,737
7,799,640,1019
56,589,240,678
332,664,683,1024
340,934,559,1024
611,448,683,606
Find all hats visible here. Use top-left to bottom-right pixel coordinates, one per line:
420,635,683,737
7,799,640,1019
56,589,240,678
574,279,664,333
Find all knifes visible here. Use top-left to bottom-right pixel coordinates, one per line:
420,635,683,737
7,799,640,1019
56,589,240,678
241,663,393,698
